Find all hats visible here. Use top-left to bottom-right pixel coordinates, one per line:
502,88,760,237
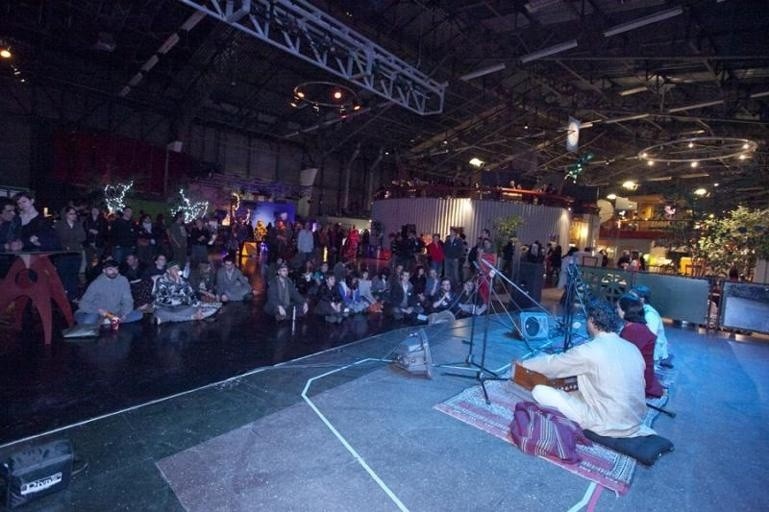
166,260,181,270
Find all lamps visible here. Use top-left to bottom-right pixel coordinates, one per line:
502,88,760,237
635,121,758,176
288,74,363,124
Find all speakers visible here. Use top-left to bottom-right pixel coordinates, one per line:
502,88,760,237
510,261,543,309
515,312,549,340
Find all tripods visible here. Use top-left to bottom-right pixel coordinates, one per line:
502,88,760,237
439,287,510,405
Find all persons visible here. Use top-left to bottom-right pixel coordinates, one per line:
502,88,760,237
615,294,665,398
561,246,646,272
519,301,658,439
629,285,669,362
1,190,561,330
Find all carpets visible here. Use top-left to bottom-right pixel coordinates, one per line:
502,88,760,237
150,306,769,512
428,331,675,500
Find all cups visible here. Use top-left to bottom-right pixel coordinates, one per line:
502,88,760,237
112,317,119,330
